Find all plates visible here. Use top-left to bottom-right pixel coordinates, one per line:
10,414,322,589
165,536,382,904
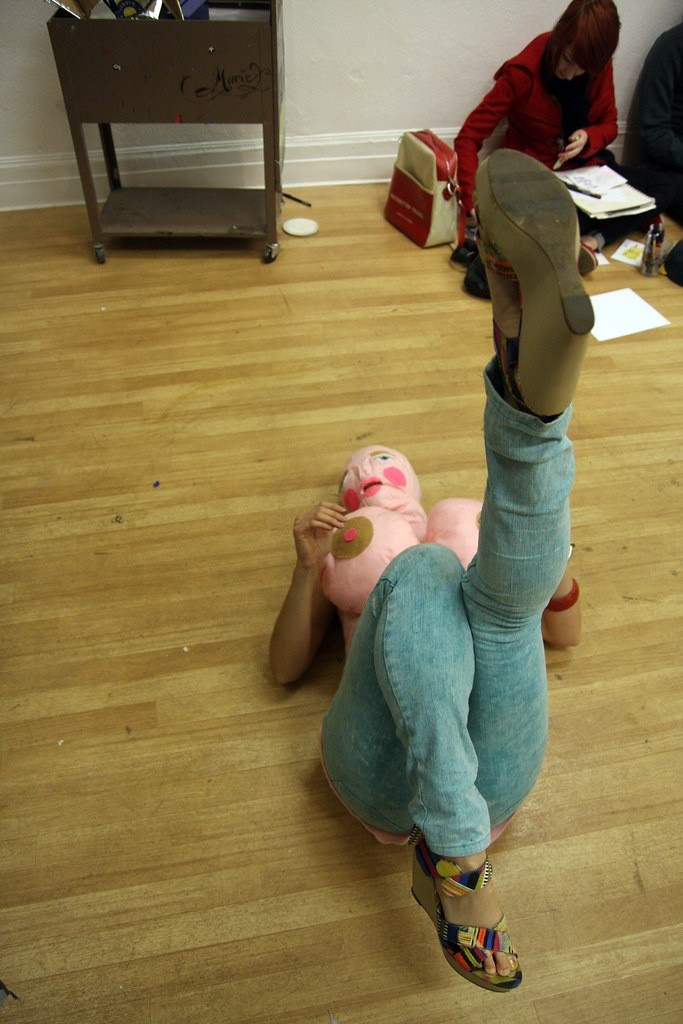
283,218,317,236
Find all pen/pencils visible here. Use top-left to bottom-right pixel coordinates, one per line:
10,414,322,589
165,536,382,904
283,193,312,206
641,222,666,276
561,181,602,199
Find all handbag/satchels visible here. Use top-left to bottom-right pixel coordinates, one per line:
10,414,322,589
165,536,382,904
384,129,458,248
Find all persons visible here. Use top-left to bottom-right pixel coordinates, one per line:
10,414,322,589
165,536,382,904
453,1,660,276
267,148,595,994
623,21,683,288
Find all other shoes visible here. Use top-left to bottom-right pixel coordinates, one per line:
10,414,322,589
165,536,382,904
577,241,598,277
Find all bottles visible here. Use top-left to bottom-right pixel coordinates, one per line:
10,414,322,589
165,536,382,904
640,219,666,277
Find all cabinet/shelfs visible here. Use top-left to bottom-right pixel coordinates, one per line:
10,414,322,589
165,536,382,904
44,0,282,263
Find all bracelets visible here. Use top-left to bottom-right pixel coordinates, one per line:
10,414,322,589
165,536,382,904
546,577,580,613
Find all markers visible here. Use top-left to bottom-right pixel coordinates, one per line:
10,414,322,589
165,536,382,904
553,135,578,170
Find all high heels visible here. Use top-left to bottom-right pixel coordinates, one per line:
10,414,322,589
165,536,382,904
407,825,523,993
476,148,595,417
